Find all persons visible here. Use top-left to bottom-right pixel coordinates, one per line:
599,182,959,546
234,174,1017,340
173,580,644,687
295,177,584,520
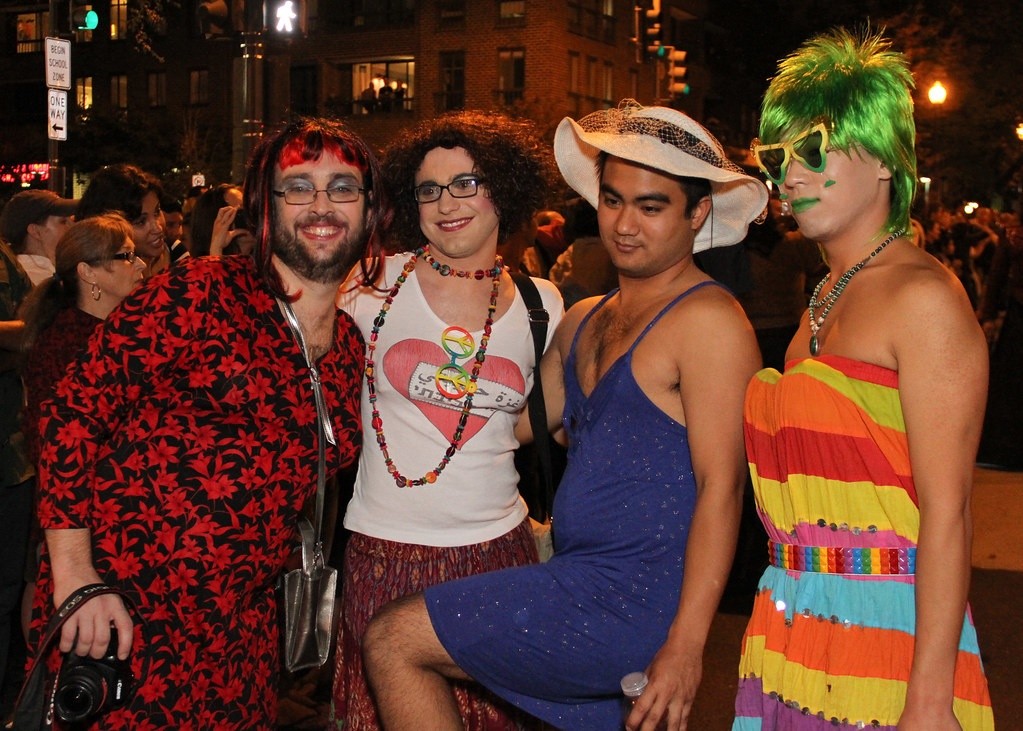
394,81,405,115
0,164,1023,715
361,82,376,115
363,101,764,731
378,78,393,115
325,111,570,731
20,117,363,731
731,34,995,731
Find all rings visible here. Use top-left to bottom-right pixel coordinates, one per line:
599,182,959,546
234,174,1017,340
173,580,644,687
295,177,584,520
218,212,223,216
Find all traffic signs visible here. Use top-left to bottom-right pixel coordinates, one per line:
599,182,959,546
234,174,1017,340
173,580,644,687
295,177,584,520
47,89,67,142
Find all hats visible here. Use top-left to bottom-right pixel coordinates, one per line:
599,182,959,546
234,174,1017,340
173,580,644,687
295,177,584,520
553,97,769,255
0,190,79,240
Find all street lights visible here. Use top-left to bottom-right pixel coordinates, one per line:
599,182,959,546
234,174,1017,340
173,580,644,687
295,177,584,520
927,80,948,215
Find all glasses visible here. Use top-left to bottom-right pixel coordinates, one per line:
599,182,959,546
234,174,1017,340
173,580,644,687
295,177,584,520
271,182,369,205
86,248,139,264
412,177,488,203
750,114,838,186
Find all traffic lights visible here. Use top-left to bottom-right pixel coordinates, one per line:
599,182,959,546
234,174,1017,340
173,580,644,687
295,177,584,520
49,0,99,41
635,1,665,63
668,49,691,97
262,0,308,40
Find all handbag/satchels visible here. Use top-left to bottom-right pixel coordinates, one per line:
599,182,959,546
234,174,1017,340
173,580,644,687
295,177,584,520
505,261,569,523
274,520,337,672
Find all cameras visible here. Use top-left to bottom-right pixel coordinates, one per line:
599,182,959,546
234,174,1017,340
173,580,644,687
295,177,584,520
53,628,142,731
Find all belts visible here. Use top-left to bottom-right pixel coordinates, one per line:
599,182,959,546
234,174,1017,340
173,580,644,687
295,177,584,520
768,538,916,574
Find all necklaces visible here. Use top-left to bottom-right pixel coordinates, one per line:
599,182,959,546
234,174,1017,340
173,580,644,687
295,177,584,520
807,230,901,356
366,244,507,488
